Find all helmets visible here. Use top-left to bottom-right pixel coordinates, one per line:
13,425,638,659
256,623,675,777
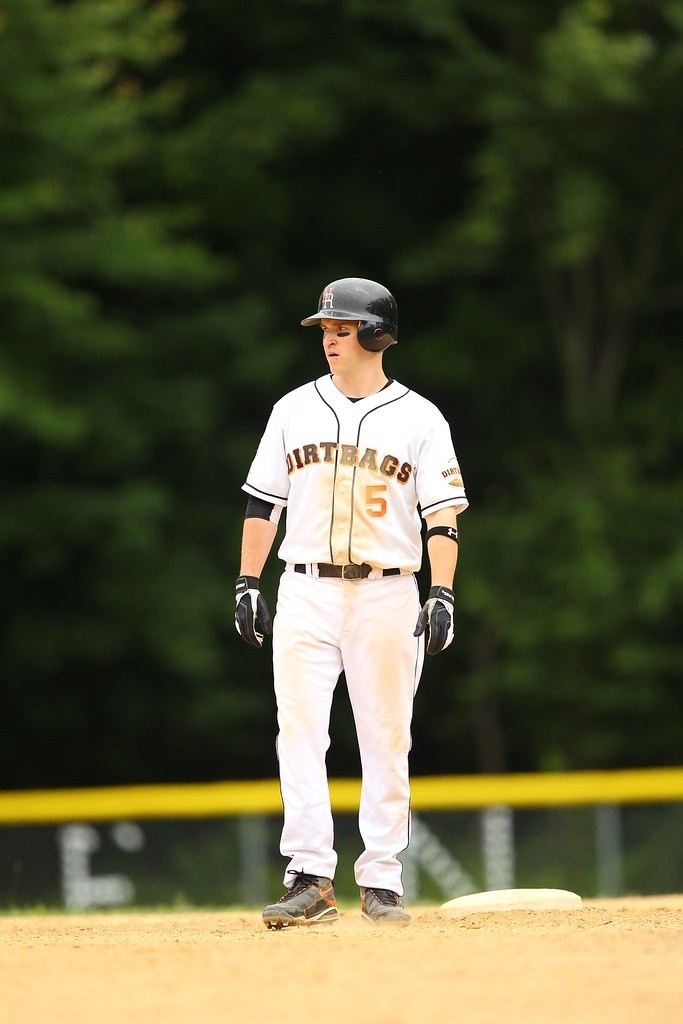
300,278,400,354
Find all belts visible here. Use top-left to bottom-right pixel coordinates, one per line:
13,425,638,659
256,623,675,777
294,562,401,580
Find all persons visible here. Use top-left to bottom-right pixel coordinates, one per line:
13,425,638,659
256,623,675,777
235,277,469,931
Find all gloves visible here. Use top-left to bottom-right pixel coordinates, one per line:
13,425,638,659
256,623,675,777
234,575,271,649
414,585,456,656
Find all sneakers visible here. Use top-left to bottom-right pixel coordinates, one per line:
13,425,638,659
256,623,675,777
359,886,413,929
262,868,340,928
